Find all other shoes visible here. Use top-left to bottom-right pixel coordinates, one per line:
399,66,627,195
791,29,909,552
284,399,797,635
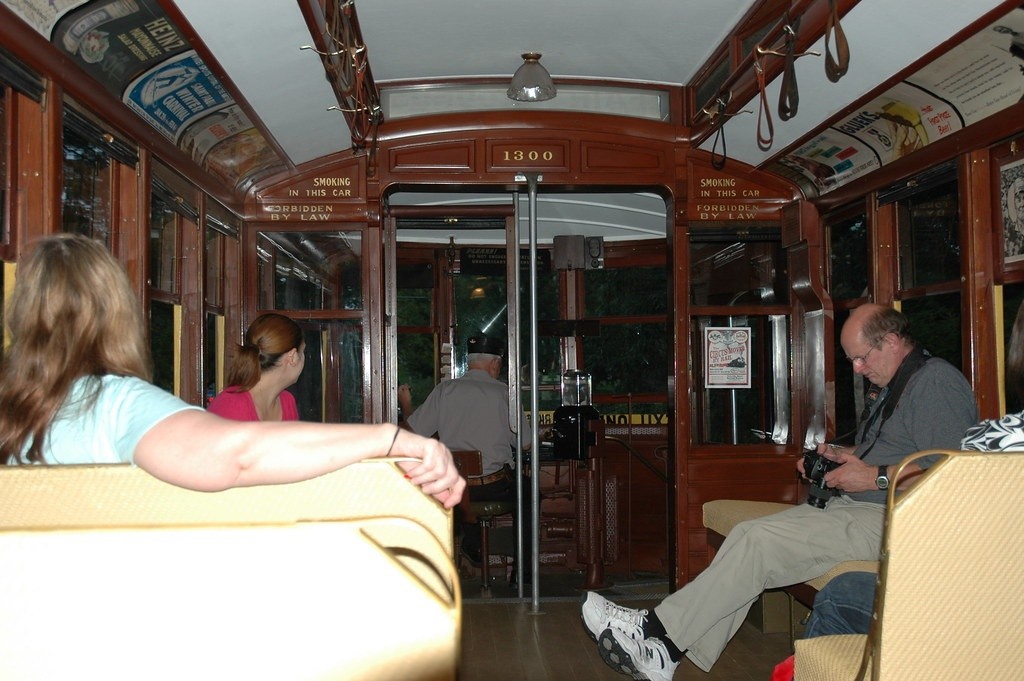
509,570,547,589
460,536,482,568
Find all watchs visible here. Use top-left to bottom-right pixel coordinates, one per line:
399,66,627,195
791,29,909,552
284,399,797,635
876,466,889,490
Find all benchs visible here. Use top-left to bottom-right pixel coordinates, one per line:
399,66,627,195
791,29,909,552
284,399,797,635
1,461,464,681
704,451,1024,681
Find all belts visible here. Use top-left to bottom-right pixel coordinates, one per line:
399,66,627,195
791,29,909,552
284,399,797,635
467,469,506,486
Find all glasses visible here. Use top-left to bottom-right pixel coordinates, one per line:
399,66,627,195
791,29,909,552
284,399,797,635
845,331,899,366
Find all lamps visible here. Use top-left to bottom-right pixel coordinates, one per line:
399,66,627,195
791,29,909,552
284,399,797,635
506,53,558,103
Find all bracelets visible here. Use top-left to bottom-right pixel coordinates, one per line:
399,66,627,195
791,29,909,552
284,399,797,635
387,427,400,456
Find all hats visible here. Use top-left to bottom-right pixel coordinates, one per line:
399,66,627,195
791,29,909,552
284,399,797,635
467,336,509,362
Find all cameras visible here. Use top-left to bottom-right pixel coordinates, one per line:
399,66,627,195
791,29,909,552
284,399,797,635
800,448,844,509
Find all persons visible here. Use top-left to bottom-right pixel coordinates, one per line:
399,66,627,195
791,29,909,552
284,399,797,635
0,235,467,510
207,313,305,421
400,334,540,589
579,305,979,681
772,306,1024,681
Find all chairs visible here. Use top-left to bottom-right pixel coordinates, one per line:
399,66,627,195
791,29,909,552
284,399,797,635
451,450,518,599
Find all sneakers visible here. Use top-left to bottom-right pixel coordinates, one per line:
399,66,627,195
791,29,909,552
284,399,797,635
597,627,680,681
578,590,648,645
